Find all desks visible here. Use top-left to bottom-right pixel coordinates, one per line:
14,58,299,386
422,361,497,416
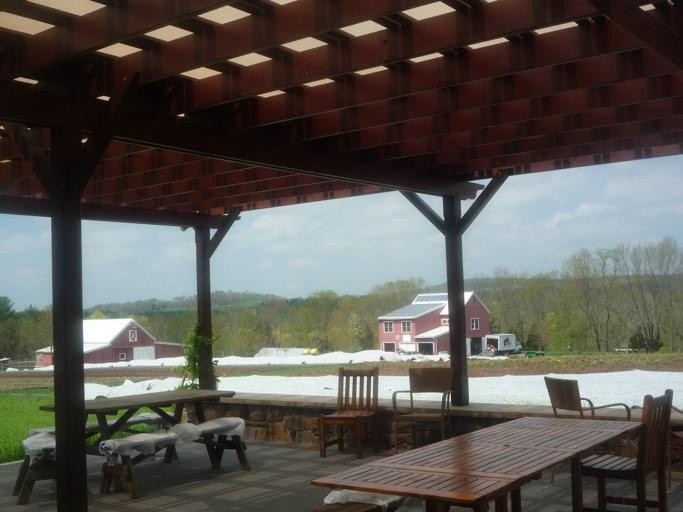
38,390,237,492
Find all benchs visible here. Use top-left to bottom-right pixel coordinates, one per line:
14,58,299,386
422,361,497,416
99,416,251,500
30,413,179,461
13,436,58,505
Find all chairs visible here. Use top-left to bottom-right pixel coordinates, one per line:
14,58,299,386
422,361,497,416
544,376,631,484
570,389,673,511
392,366,455,455
319,366,378,459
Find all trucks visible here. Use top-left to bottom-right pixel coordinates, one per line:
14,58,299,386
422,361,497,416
484,333,522,355
